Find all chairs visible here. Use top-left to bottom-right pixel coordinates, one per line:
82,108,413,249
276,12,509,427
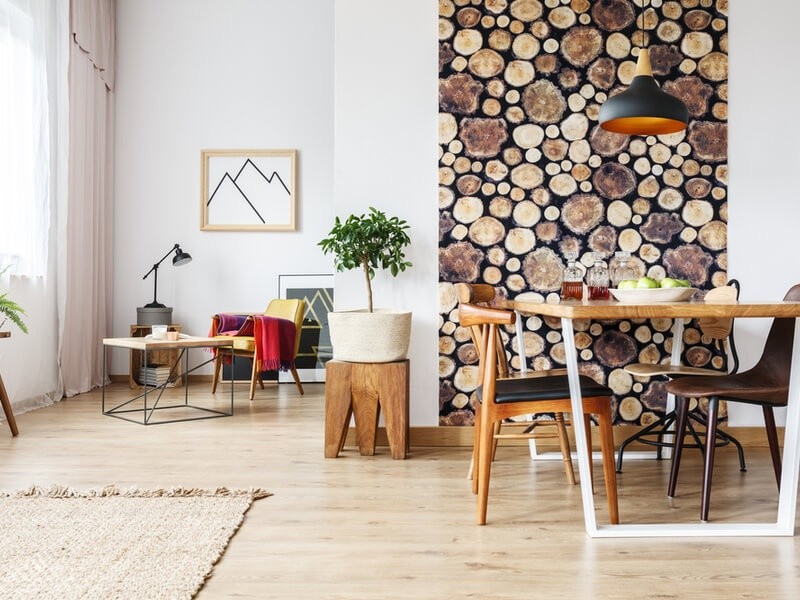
211,299,304,399
664,284,800,524
453,282,620,525
617,278,747,473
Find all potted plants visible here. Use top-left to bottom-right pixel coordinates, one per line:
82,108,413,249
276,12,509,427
317,206,412,364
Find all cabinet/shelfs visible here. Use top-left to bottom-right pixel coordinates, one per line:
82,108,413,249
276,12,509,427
130,324,182,390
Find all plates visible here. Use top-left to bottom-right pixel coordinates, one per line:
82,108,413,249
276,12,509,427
608,288,699,301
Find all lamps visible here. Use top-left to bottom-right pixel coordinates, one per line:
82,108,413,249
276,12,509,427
599,0,688,136
142,243,193,308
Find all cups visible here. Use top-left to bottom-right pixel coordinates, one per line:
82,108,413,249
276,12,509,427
152,325,167,338
167,332,179,341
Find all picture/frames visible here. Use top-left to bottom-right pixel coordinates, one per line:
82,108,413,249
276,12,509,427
278,274,334,384
200,149,296,232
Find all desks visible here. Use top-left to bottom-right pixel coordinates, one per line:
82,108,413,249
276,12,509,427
102,333,234,426
325,359,410,460
497,300,800,538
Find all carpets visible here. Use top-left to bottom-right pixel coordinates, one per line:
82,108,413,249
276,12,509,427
0,485,274,600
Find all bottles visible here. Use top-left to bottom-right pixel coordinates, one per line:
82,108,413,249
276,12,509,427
562,251,583,300
588,252,609,300
614,252,634,288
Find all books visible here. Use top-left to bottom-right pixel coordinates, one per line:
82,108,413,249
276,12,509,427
139,363,176,387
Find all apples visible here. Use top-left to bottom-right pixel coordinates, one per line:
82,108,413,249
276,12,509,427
618,277,691,289
